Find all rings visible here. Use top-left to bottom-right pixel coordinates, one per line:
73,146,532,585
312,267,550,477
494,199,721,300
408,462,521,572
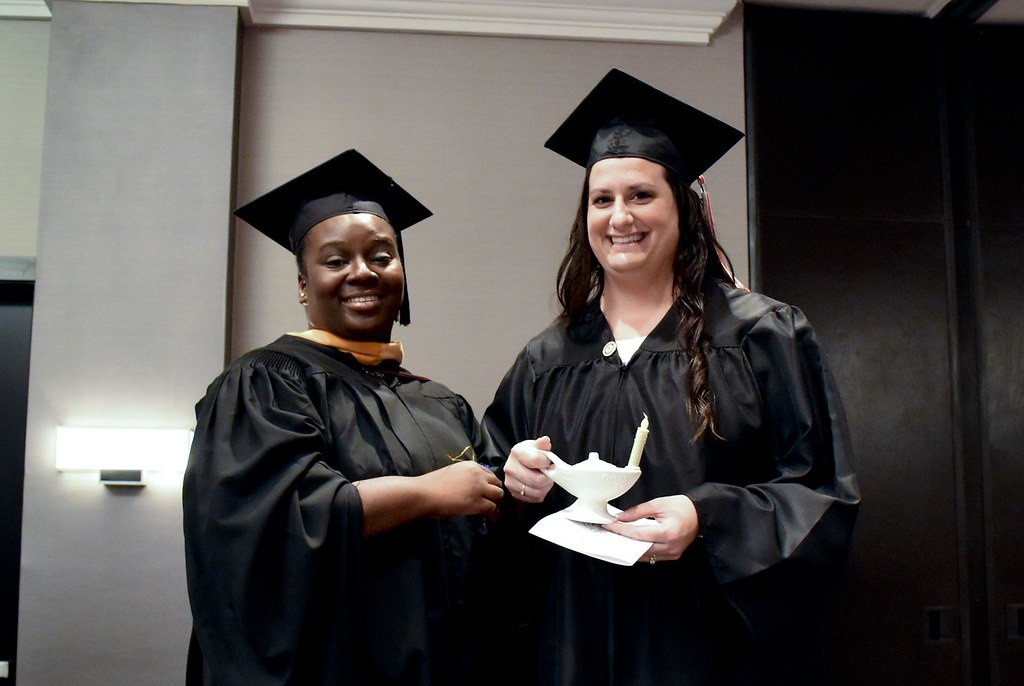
520,484,526,496
650,554,657,566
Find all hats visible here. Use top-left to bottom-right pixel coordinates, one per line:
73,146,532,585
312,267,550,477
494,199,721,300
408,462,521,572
233,148,433,325
544,66,751,294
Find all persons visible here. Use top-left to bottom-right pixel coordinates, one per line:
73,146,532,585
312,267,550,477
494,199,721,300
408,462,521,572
479,66,861,686
182,148,504,686
